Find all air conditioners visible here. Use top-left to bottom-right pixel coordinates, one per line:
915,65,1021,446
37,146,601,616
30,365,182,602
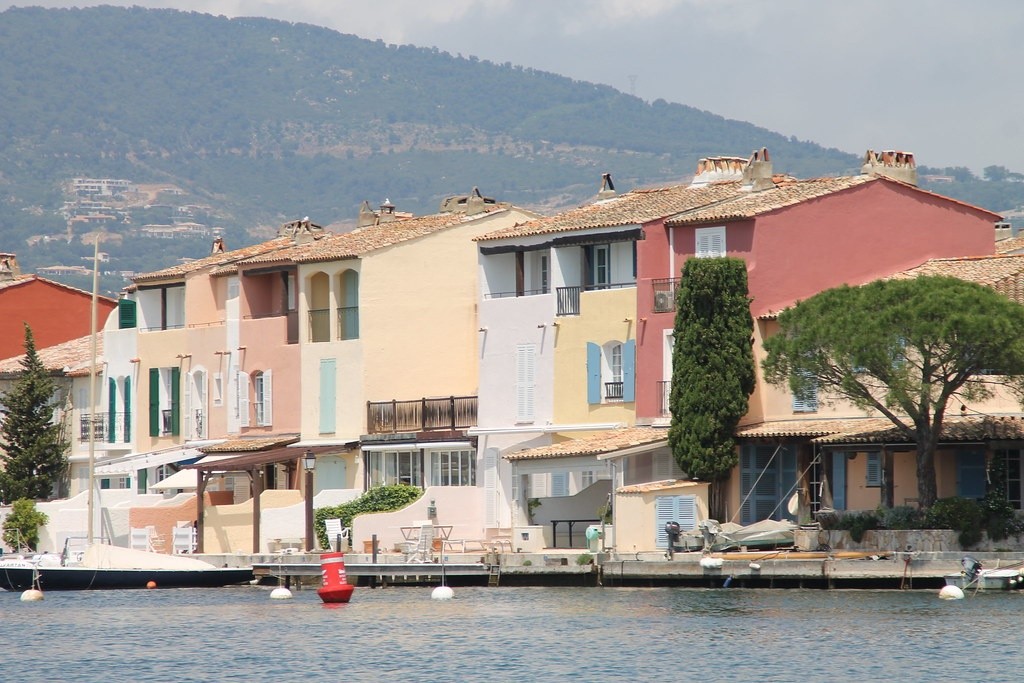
654,291,674,313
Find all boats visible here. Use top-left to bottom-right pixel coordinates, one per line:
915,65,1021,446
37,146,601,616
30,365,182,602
943,556,1020,591
665,521,800,553
1,541,254,592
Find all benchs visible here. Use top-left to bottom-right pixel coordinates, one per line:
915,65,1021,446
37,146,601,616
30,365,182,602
441,537,486,553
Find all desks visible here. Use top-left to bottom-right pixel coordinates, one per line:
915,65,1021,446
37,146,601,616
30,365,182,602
550,519,601,548
268,535,306,553
400,525,454,553
